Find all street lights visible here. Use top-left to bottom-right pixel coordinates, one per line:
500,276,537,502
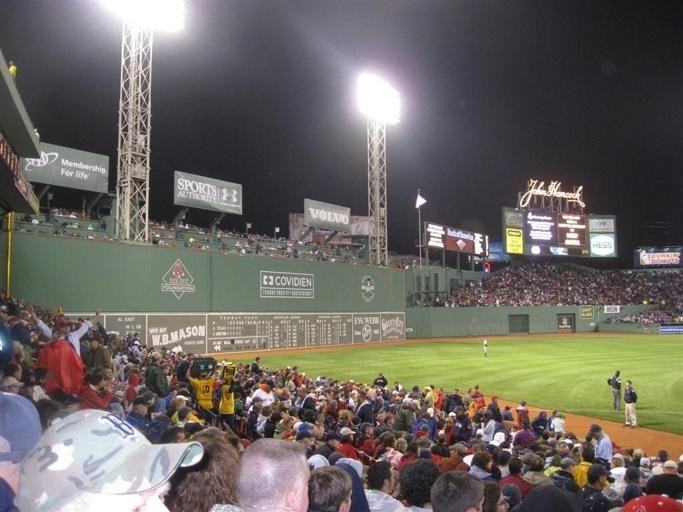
95,0,190,247
353,69,402,266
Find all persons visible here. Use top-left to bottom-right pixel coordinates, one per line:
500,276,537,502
426,261,683,324
1,291,683,512
3,205,408,270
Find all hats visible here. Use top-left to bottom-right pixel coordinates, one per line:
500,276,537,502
348,382,680,512
12,408,205,512
131,367,345,512
0,390,43,463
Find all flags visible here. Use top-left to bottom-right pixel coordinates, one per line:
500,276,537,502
414,195,427,208
